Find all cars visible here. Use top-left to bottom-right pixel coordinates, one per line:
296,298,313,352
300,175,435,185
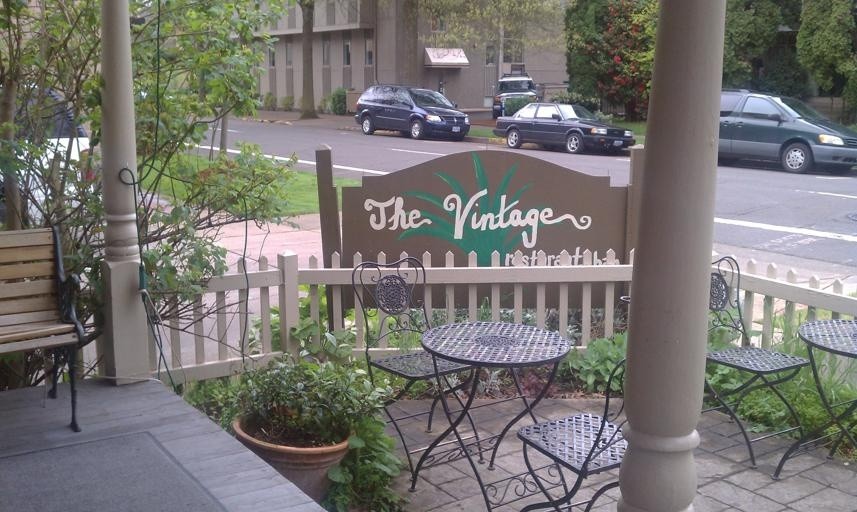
1,82,94,229
717,87,857,173
492,101,637,155
353,84,471,140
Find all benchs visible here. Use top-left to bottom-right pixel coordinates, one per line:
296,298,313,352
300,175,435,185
0,226,102,432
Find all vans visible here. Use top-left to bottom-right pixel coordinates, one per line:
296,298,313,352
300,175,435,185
491,73,538,119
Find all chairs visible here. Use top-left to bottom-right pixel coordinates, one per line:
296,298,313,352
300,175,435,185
701,257,811,470
518,360,630,512
352,257,485,480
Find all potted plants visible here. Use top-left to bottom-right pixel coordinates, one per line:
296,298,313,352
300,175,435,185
231,320,408,512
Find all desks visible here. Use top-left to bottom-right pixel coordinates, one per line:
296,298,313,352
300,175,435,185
772,320,857,481
409,323,569,512
